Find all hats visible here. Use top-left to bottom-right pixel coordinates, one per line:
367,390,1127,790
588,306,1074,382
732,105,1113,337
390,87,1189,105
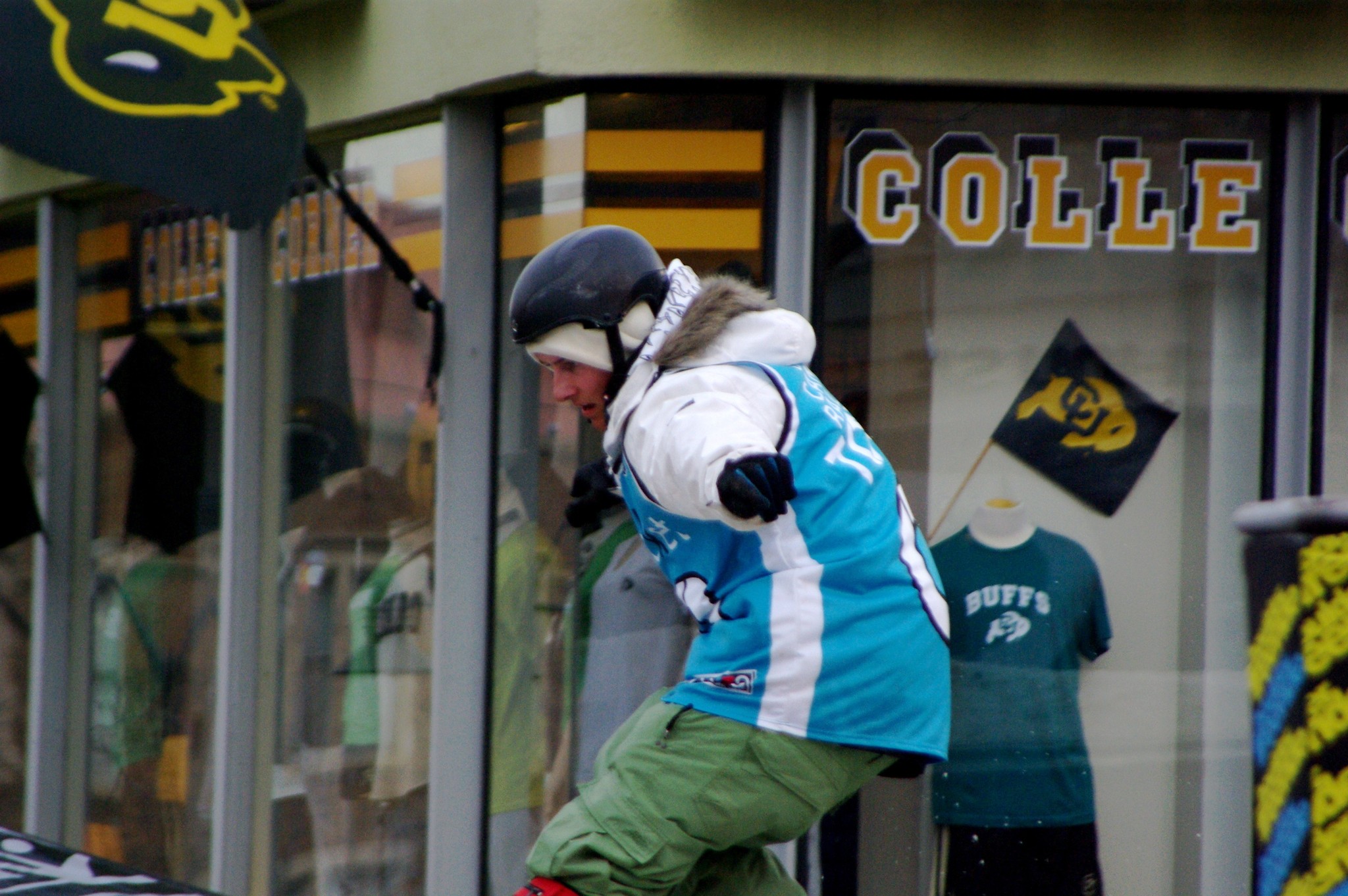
526,303,655,373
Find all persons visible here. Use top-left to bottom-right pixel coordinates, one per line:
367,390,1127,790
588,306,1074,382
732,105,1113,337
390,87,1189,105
96,468,692,896
505,224,955,896
930,494,1104,896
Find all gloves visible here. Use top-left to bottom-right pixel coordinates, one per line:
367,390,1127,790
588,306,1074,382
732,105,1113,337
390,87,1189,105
563,460,628,540
717,455,797,522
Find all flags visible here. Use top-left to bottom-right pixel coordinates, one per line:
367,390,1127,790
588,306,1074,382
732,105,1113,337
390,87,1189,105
992,317,1182,516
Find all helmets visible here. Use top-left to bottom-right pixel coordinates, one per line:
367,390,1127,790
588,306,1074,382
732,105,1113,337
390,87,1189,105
509,225,668,343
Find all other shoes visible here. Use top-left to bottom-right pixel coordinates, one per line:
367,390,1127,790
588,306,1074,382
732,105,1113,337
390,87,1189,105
84,824,124,864
